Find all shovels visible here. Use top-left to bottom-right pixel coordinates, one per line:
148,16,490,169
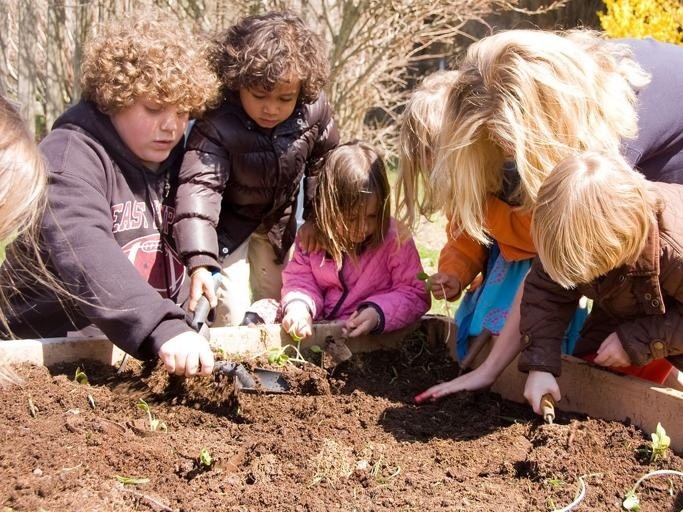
212,360,291,394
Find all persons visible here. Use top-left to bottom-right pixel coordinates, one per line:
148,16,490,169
280,143,431,338
412,27,683,406
519,153,683,414
380,70,538,301
0,96,48,269
173,12,341,325
35,8,222,379
